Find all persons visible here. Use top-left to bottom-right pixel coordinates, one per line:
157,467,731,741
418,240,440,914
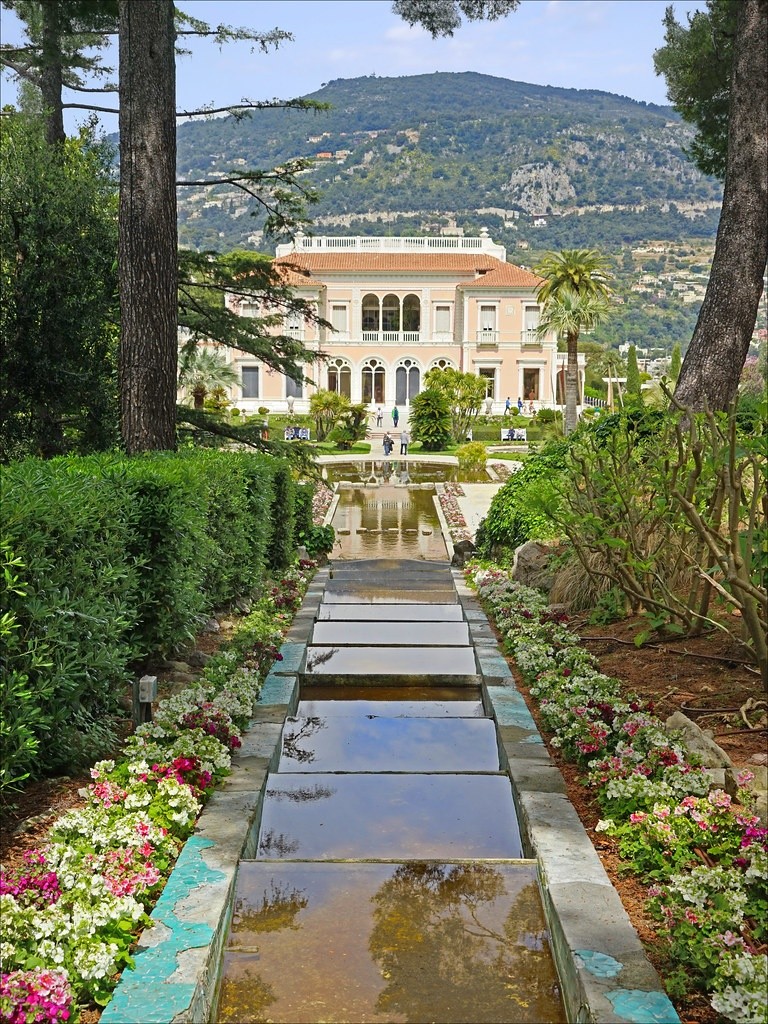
508,425,515,441
391,407,399,427
529,402,535,415
300,425,309,441
516,426,525,441
382,431,395,456
528,388,536,400
400,430,410,456
289,423,305,440
518,397,524,412
504,396,511,415
283,426,293,441
375,407,383,428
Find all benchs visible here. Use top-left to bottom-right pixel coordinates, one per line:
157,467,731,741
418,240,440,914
500,428,528,442
462,429,472,442
284,427,311,441
